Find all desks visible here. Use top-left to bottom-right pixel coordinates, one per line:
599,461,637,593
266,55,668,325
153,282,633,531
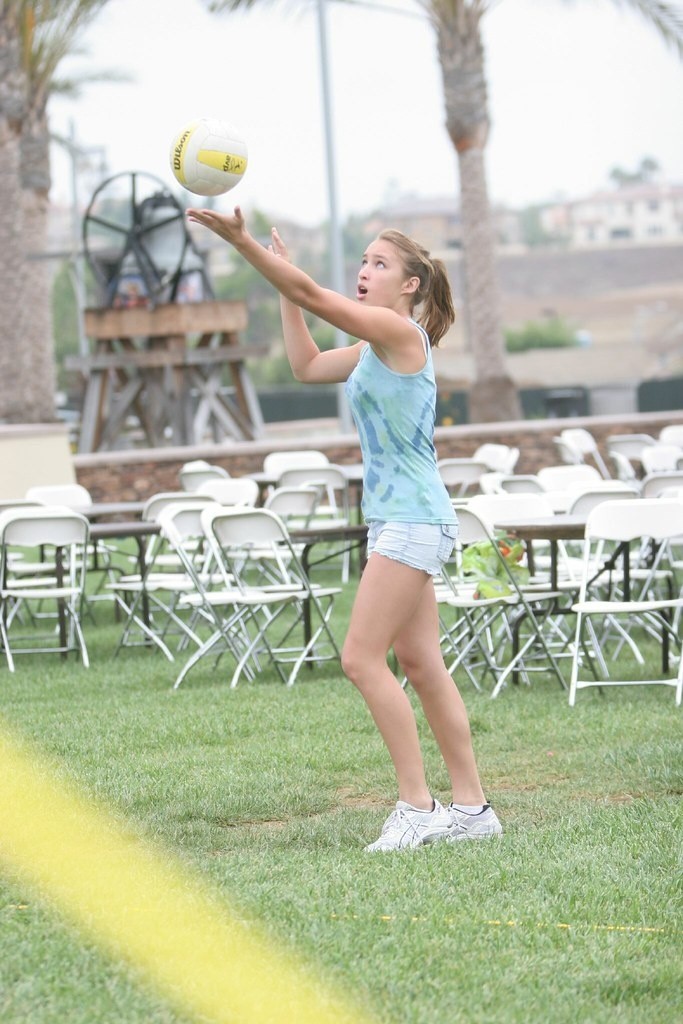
3,521,161,657
490,521,676,685
239,467,367,575
280,523,369,669
77,501,149,602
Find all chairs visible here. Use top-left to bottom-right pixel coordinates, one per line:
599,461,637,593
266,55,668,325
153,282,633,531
0,425,683,709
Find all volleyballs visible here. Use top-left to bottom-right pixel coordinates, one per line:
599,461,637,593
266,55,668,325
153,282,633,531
166,112,252,198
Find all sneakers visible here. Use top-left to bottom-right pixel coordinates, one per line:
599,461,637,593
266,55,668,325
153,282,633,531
445,801,503,840
362,799,456,853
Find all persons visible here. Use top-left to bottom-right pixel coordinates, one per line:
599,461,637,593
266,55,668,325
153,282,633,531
185,205,504,852
